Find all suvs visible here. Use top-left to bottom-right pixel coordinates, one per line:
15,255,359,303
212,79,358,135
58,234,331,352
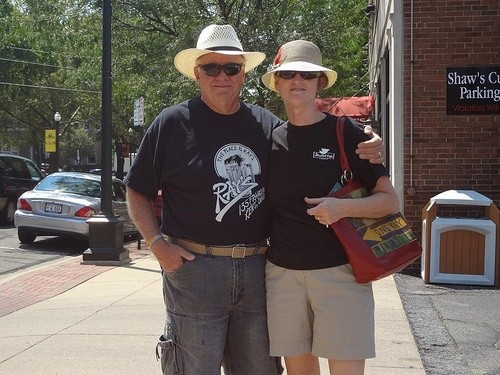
0,151,45,227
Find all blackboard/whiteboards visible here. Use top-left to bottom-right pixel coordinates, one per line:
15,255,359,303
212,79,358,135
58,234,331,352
445,66,500,115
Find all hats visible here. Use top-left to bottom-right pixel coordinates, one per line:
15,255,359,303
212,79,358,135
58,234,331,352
260,40,338,91
174,23,267,81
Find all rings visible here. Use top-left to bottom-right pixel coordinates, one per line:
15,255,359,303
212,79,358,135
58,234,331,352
378,151,382,158
317,216,321,221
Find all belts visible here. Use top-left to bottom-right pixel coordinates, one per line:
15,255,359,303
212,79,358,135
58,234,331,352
165,236,268,258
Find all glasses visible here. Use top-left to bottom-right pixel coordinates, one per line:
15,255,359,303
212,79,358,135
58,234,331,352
277,71,323,79
196,62,243,77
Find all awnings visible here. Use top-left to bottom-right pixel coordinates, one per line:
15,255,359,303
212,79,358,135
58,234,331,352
314,96,373,118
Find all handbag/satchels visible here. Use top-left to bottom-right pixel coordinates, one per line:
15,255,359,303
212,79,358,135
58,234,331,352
325,114,423,284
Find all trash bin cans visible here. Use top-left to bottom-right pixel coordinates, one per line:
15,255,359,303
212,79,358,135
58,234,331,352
421,190,500,288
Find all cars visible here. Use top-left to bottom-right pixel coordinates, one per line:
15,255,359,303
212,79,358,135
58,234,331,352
14,169,162,254
41,162,62,175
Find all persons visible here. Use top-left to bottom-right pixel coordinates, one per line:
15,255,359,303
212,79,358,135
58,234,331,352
264,40,400,375
122,25,385,375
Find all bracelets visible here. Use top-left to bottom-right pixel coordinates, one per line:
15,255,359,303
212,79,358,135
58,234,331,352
147,234,162,248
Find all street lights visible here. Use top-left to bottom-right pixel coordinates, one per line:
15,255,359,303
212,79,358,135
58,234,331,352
54,111,62,172
128,128,133,166
